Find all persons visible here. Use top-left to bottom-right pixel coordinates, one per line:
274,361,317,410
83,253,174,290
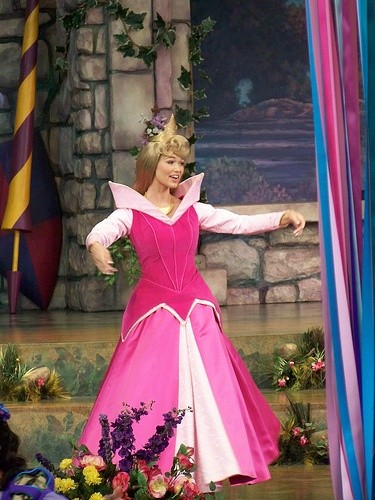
73,114,305,492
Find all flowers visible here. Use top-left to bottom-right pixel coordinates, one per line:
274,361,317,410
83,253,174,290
34,400,224,500
126,106,168,155
255,342,330,466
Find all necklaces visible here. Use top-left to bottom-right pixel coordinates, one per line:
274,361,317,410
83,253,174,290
144,194,176,216
